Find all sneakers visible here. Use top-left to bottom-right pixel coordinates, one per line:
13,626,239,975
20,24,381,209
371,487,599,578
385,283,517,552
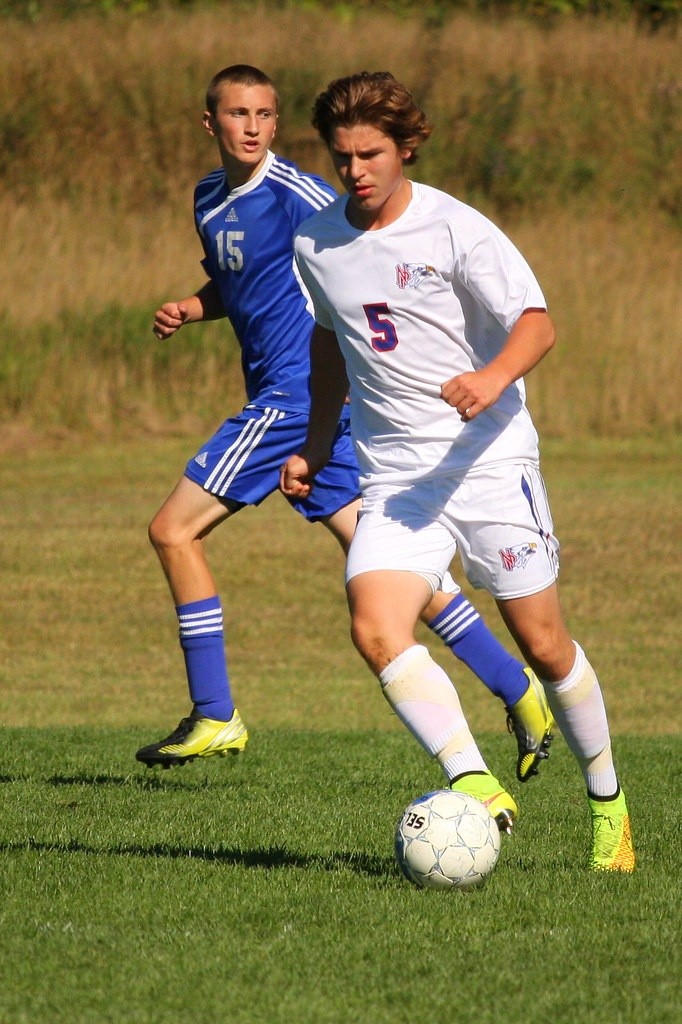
505,671,553,785
133,710,249,767
451,775,518,832
587,781,635,874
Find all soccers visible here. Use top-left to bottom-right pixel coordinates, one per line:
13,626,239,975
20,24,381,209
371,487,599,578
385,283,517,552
395,788,502,887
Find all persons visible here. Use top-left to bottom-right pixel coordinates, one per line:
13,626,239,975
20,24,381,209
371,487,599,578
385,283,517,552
277,70,633,874
134,65,557,785
